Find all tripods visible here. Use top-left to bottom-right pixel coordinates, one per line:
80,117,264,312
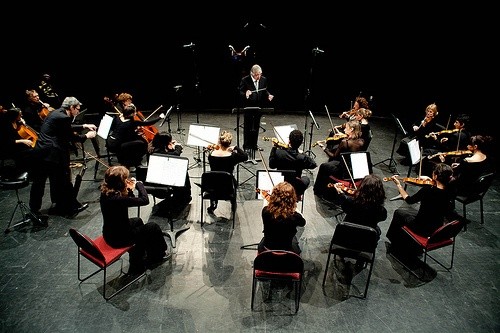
304,111,321,157
188,82,209,170
372,114,408,175
144,153,190,248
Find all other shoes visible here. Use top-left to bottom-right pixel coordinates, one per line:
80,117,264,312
253,146,263,151
244,148,250,155
276,284,285,289
408,247,416,265
400,240,409,257
231,202,237,211
128,261,145,274
47,206,76,218
355,260,364,268
287,282,293,290
31,206,42,215
145,255,161,264
207,204,217,214
335,254,346,271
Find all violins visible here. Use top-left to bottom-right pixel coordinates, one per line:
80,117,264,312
312,133,348,149
421,116,434,127
254,187,271,203
382,176,435,186
425,128,465,138
262,136,291,150
338,109,357,119
427,150,473,159
328,121,360,132
327,182,357,198
125,176,174,193
206,144,220,151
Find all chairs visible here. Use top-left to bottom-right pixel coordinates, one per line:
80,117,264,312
321,167,362,211
322,220,377,299
251,247,304,316
296,177,304,213
90,131,118,181
69,229,147,301
137,186,180,220
387,123,494,278
201,171,238,229
106,135,147,171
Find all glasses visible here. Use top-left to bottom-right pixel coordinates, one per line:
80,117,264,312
76,108,80,111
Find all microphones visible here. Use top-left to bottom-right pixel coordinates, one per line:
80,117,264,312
312,49,324,53
183,44,195,47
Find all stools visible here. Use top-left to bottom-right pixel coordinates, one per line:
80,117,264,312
0,171,48,235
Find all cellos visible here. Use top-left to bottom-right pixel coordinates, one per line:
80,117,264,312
26,90,55,121
104,97,149,147
114,94,160,141
12,103,39,148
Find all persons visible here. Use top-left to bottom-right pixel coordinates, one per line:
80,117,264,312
385,163,455,261
337,174,387,273
107,93,165,164
207,130,248,212
396,103,437,166
258,182,306,255
327,97,372,150
99,166,168,275
269,130,316,195
147,131,191,198
415,114,492,210
29,97,97,216
0,90,39,147
239,64,274,101
318,120,365,162
